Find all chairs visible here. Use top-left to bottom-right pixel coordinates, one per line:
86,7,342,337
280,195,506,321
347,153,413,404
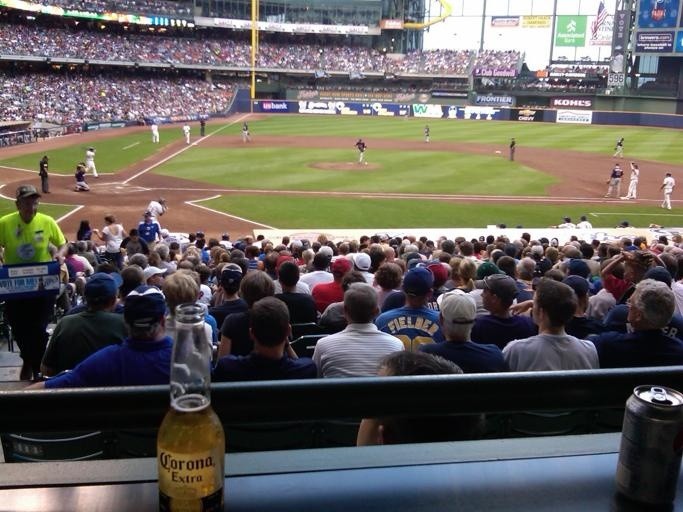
1,310,683,461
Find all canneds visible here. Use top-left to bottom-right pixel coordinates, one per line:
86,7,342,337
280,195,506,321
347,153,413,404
616,384,683,504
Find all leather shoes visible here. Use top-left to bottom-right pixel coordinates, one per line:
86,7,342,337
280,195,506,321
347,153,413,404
33,368,43,382
20,365,32,380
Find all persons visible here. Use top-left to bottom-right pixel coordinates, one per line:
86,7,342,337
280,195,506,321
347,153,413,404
612,136,626,160
625,161,641,200
355,351,472,447
658,172,677,211
38,155,51,194
81,145,100,179
211,295,318,383
147,197,166,217
2,76,251,147
352,137,369,167
508,136,517,162
26,286,212,389
586,278,683,370
424,125,430,143
1,185,69,380
415,288,509,373
0,0,630,94
72,162,90,191
502,277,602,370
312,282,404,378
603,162,625,199
49,212,683,382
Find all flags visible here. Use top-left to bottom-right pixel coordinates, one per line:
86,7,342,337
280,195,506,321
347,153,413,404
593,3,608,38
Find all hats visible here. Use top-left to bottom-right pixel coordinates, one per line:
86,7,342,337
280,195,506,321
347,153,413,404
83,272,123,303
624,245,639,251
473,274,519,300
143,266,167,280
402,263,477,324
319,245,371,274
15,185,41,198
123,284,167,327
561,274,596,295
220,263,242,285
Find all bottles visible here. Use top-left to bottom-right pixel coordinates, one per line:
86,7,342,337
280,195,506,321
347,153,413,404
155,302,226,511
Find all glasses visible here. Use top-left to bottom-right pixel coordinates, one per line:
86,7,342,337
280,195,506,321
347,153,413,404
482,276,490,289
